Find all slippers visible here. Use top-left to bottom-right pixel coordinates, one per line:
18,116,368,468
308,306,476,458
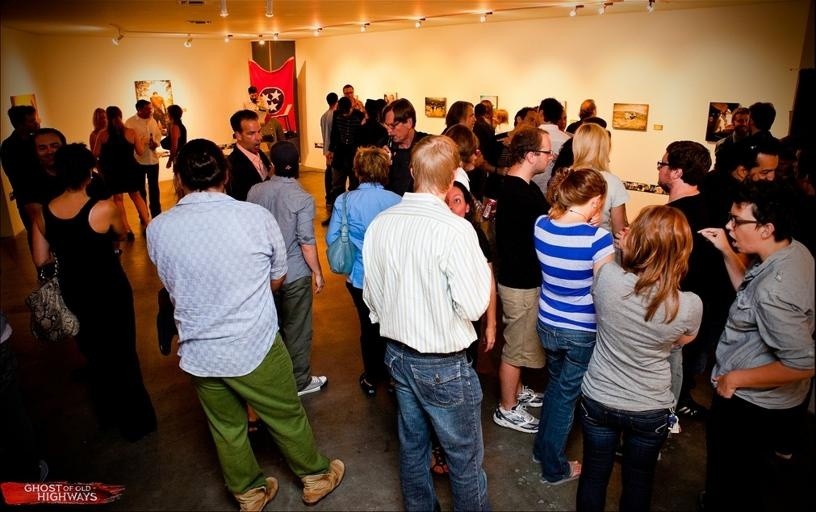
533,448,581,487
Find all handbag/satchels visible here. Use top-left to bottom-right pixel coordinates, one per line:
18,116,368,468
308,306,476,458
326,191,358,275
25,255,80,340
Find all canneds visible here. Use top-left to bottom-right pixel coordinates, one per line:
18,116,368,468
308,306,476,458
483,199,496,218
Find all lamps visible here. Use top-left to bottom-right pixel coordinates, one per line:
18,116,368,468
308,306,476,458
110,1,657,50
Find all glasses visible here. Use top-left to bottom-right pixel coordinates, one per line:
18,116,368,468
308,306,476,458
728,212,761,229
383,121,401,130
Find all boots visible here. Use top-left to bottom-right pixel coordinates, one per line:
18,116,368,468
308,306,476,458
301,458,346,506
233,476,280,512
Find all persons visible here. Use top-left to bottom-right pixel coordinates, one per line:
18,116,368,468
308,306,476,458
574,203,704,511
146,137,349,510
695,180,816,512
359,132,493,512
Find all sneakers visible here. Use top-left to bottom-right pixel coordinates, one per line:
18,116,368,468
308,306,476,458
513,383,543,407
359,371,377,396
297,374,328,398
492,403,540,435
675,388,709,422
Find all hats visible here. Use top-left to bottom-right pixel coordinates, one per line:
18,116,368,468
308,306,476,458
365,98,387,116
270,141,300,176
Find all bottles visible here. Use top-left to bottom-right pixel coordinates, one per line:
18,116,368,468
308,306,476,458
386,137,394,166
149,133,154,146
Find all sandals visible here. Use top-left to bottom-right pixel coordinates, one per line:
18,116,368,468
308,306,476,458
429,445,449,474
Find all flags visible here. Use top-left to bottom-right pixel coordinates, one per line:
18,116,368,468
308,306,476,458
246,57,297,135
